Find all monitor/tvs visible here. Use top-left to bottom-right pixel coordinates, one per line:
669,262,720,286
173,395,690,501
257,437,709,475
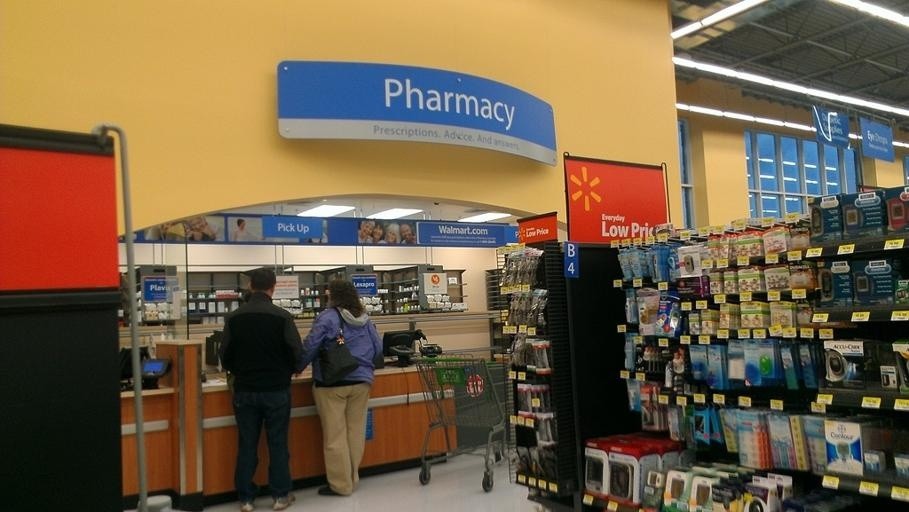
118,346,148,387
383,330,417,366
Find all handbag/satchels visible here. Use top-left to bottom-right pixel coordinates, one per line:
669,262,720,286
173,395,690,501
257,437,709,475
318,334,360,386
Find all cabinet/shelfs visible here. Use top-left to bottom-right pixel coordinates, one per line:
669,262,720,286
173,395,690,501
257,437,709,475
124,263,469,325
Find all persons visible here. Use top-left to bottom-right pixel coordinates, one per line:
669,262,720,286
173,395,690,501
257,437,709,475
218,268,303,512
296,279,383,497
357,220,417,245
232,219,264,241
186,214,216,243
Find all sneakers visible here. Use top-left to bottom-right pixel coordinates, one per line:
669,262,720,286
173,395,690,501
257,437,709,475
240,501,255,512
319,486,340,495
273,494,295,510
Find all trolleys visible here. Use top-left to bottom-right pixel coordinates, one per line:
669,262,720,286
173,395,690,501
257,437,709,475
405,343,514,495
